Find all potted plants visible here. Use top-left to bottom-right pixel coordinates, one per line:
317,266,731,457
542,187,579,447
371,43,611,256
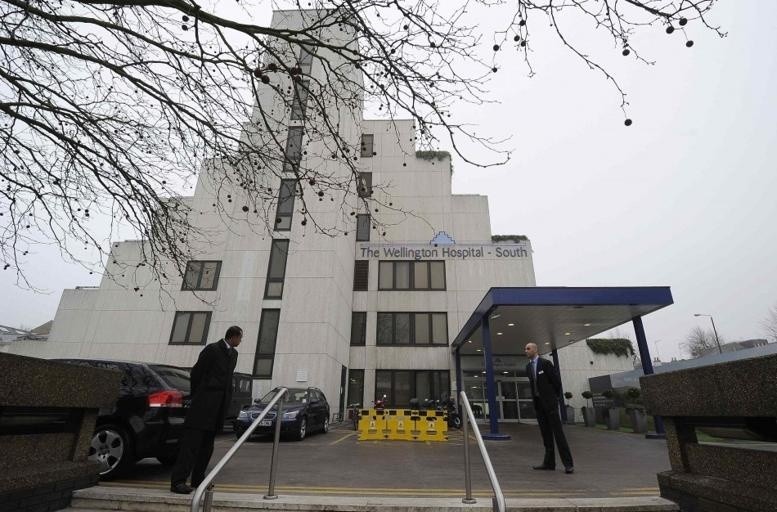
562,386,649,434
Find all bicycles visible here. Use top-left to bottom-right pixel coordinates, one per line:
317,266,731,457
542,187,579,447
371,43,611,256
349,403,361,431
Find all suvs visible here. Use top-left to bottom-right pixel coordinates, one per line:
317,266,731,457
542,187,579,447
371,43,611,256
233,386,331,441
224,372,252,426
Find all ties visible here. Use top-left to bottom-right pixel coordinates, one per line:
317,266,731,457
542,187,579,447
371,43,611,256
531,361,536,383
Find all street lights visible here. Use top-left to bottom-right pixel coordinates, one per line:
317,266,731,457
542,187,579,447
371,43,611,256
694,313,723,354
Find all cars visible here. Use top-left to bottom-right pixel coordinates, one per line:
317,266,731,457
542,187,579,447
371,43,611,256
41,358,191,480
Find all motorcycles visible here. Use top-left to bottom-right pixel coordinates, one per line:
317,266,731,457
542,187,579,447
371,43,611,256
427,397,462,429
371,393,388,418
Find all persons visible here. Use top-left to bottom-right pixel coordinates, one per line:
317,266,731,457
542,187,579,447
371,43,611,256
524,342,574,472
169,325,242,493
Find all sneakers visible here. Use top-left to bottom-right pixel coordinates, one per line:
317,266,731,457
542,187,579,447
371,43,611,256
565,466,575,474
170,482,193,494
532,462,556,471
191,479,214,489
